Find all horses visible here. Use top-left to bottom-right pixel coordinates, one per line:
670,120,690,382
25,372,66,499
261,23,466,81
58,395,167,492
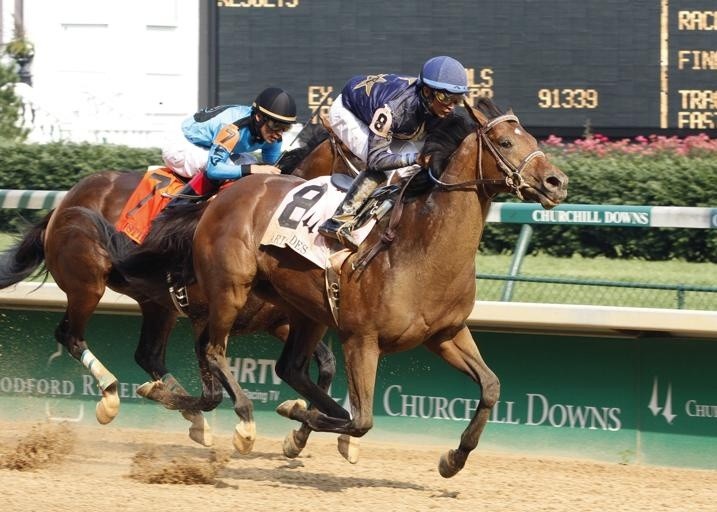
66,93,570,479
0,123,362,460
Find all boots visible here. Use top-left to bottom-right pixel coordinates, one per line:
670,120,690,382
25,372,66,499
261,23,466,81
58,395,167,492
166,183,206,211
318,168,389,253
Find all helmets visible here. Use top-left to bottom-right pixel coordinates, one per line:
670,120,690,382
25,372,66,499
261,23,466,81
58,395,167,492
252,87,297,124
417,55,472,94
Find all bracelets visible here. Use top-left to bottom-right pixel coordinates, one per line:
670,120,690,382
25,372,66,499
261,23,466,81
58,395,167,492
241,164,250,176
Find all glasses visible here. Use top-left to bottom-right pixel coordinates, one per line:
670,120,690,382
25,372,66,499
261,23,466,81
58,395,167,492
251,107,292,132
419,63,465,107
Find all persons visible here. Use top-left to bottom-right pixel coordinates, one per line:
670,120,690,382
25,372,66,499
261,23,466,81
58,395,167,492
159,88,297,207
317,56,468,251
